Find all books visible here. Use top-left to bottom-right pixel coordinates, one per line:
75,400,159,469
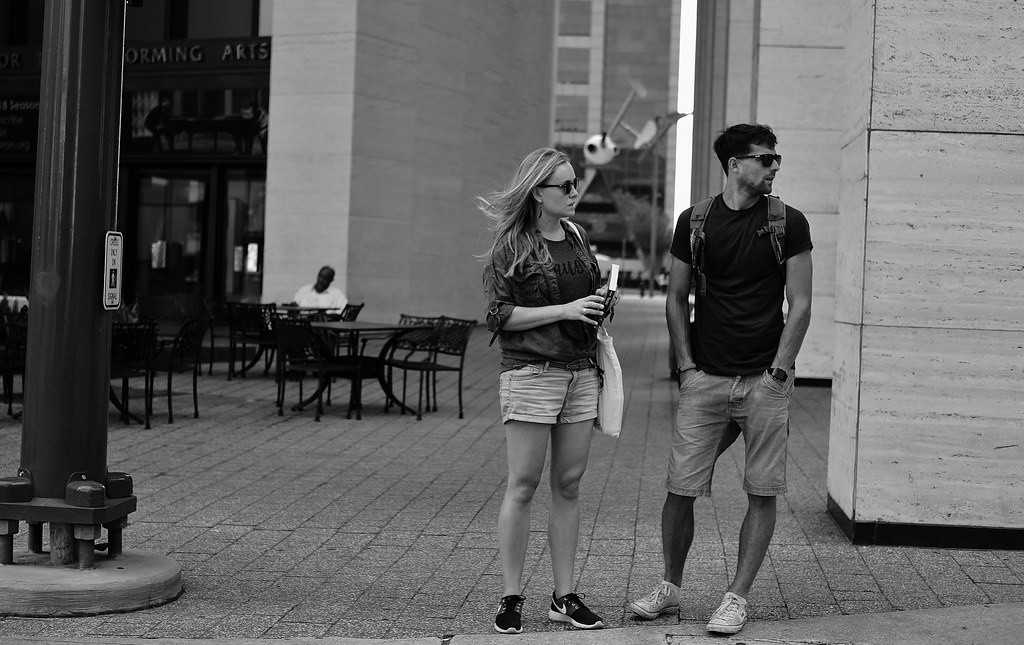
594,263,620,330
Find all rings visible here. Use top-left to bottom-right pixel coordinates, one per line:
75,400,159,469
583,308,586,315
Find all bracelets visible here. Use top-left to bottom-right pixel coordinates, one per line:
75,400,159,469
676,363,697,374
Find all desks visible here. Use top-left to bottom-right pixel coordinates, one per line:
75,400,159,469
258,306,343,375
285,320,404,418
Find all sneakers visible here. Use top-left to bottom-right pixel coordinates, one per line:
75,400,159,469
706,592,747,633
630,583,680,618
548,591,603,628
495,594,526,633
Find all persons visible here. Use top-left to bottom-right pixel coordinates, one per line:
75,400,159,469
473,147,621,634
289,266,349,381
630,124,813,635
657,267,671,296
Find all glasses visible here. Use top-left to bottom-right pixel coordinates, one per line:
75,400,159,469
737,154,782,168
538,177,580,195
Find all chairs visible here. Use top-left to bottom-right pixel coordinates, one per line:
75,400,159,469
0,300,478,423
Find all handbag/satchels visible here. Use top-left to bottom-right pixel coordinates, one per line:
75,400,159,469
593,321,624,437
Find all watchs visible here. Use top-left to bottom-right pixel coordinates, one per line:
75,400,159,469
767,367,788,382
